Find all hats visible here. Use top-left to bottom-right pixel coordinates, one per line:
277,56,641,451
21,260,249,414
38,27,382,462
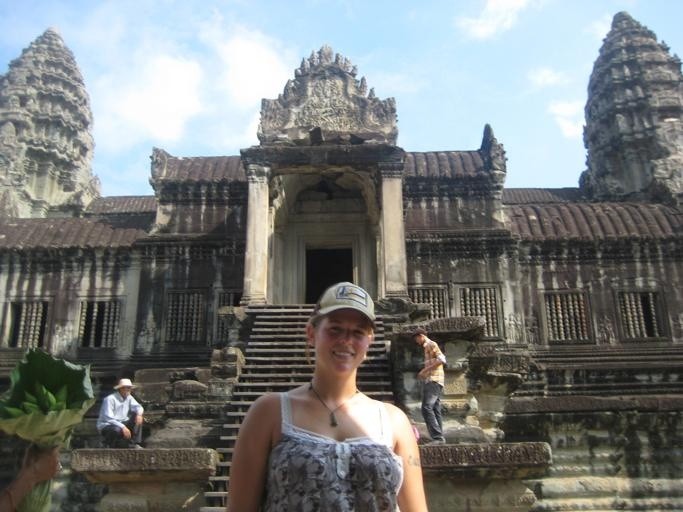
412,329,427,336
113,378,137,390
311,282,376,330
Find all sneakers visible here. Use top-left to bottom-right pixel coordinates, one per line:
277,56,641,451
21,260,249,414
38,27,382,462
428,438,446,445
99,441,110,448
129,443,141,449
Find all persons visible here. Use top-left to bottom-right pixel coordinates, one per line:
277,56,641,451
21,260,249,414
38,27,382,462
226,282,428,512
412,327,447,446
96,378,144,449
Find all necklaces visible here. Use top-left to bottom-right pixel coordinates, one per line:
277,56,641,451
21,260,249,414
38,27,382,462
308,381,359,426
